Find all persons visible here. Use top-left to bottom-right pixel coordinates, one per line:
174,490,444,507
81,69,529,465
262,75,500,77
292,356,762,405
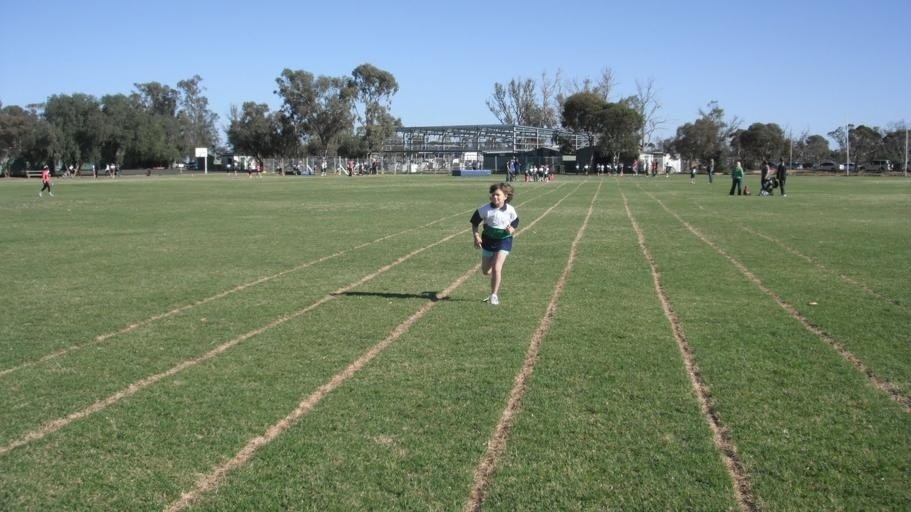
38,167,54,197
64,166,75,179
248,159,262,178
728,157,786,196
576,158,670,177
525,164,549,182
708,158,715,183
105,161,119,176
505,157,520,183
470,184,519,305
691,167,696,184
285,159,355,176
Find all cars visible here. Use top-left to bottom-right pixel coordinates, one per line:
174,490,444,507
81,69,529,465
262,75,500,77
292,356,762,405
763,159,860,172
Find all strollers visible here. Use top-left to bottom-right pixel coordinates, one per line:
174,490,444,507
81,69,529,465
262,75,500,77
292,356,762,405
757,176,780,196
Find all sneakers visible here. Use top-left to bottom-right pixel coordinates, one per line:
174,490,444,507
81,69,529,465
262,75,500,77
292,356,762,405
490,294,498,305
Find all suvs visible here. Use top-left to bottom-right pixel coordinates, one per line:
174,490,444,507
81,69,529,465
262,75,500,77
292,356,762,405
858,160,895,173
